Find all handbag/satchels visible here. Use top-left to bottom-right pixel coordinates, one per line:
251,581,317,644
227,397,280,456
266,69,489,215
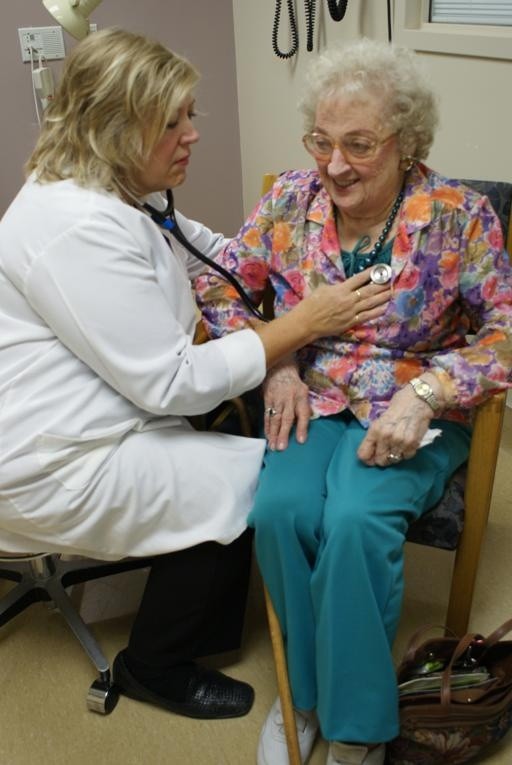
390,617,512,763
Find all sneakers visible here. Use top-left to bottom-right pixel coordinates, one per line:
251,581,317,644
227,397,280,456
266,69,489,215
256,695,319,764
325,740,385,764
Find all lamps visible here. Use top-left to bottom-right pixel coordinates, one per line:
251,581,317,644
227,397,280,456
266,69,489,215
42,0,105,42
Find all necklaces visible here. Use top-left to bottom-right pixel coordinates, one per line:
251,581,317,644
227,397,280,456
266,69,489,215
349,185,408,280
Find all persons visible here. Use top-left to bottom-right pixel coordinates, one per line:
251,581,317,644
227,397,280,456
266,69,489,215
190,33,512,763
0,25,401,719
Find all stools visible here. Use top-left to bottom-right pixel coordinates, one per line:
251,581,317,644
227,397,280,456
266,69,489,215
1,549,151,720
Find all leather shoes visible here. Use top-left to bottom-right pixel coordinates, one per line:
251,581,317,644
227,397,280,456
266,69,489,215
111,649,255,719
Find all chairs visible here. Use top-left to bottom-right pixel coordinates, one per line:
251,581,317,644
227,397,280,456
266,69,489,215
252,173,512,639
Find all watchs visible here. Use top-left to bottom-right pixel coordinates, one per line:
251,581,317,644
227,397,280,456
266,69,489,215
409,377,441,411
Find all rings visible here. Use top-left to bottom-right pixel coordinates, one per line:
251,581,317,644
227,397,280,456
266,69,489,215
355,289,362,301
268,408,282,418
355,313,360,325
387,453,401,464
263,409,270,416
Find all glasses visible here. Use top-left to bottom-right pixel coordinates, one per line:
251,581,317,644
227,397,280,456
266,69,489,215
301,124,403,166
